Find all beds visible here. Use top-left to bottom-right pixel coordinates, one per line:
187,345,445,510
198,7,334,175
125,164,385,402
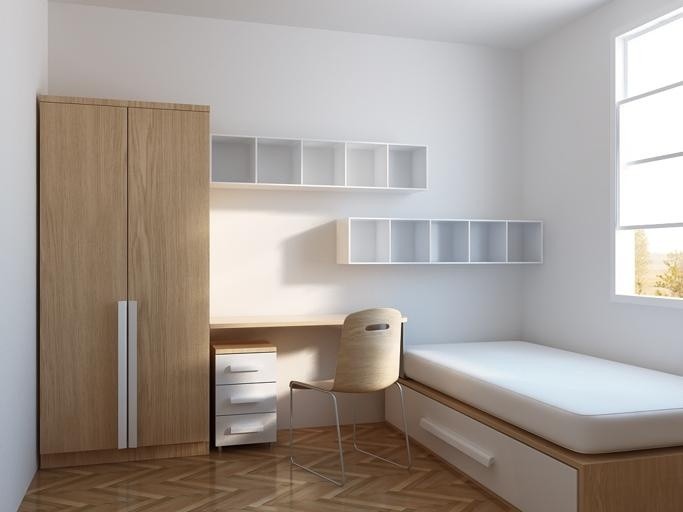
383,341,683,512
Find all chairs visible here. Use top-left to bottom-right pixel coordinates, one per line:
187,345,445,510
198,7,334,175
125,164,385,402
285,308,413,487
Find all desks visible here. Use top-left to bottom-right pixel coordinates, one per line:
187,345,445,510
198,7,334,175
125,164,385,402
209,315,412,455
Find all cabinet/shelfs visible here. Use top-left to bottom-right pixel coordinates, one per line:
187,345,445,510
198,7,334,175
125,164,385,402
36,94,216,469
209,131,546,269
